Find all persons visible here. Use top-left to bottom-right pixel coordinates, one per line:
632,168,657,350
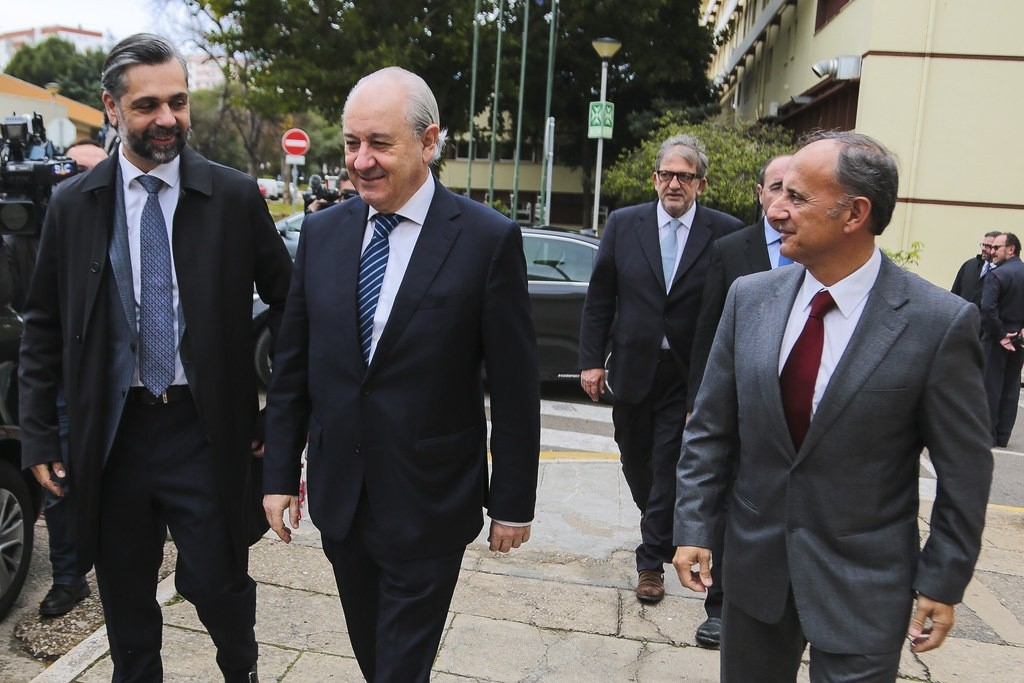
980,232,1024,449
579,138,744,601
36,142,110,620
264,66,543,683
670,133,995,683
18,31,295,683
305,171,357,215
952,230,998,302
687,154,798,649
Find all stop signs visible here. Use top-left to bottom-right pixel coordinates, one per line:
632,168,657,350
280,127,310,156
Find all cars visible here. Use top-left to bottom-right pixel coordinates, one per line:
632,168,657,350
257,177,298,201
0,232,43,623
252,224,619,407
274,210,306,265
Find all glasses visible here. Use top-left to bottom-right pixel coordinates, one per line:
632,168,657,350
991,245,1008,250
979,242,990,250
655,170,698,185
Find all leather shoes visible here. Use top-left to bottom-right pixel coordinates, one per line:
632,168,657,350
634,569,665,602
694,617,721,646
39,579,91,617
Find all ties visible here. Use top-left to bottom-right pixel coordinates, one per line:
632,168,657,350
660,218,684,293
134,174,175,398
775,239,795,268
980,262,992,276
357,213,405,369
780,292,840,454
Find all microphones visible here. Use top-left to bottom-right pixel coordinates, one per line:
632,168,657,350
309,175,323,195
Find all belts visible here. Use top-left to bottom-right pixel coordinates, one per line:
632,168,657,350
129,387,190,407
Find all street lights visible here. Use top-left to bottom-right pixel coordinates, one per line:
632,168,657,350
590,36,622,239
